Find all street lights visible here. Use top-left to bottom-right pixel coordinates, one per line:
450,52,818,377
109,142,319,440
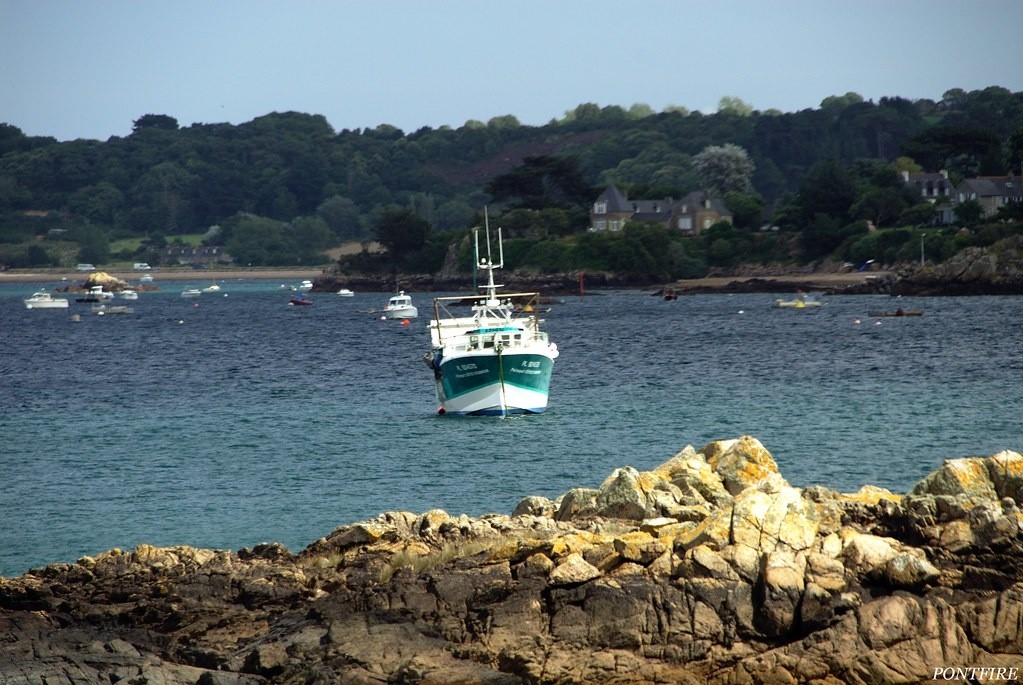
921,231,928,270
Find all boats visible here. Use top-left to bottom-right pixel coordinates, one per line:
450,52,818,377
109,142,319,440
75,284,115,303
380,290,418,320
337,289,355,298
141,273,152,283
299,279,314,291
291,296,315,306
23,288,70,310
771,298,824,310
421,204,560,417
120,286,141,300
181,283,221,298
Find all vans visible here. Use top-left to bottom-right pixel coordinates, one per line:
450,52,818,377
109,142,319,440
133,262,152,270
77,263,96,272
